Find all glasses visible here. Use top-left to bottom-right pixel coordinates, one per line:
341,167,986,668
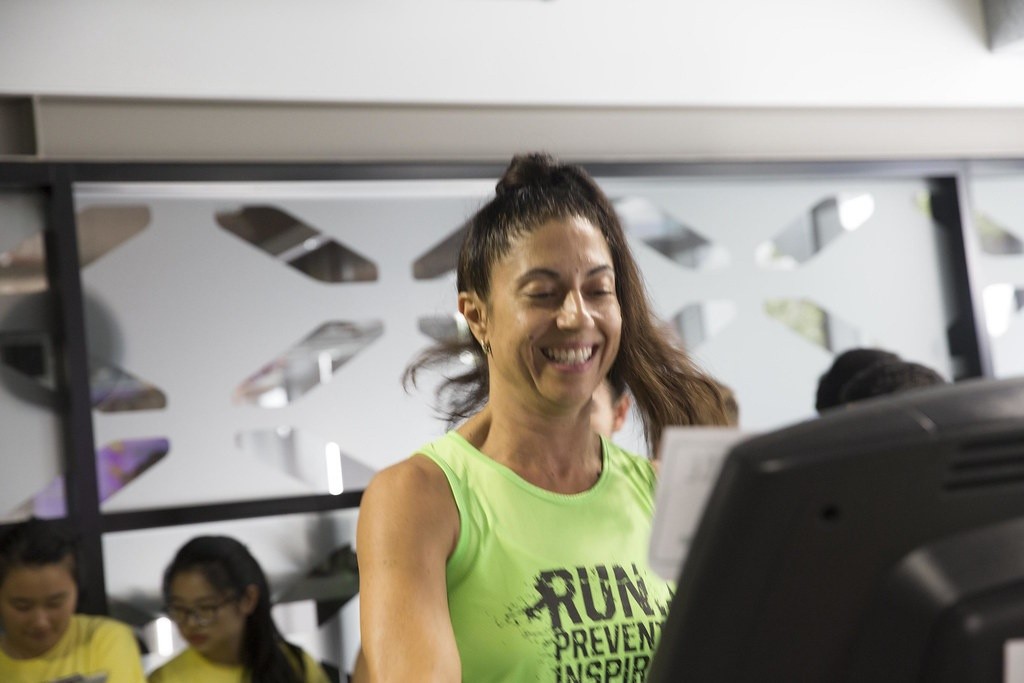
168,594,241,626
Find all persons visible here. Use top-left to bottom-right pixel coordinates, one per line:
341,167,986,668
0,521,145,683
814,350,946,415
147,535,330,683
353,154,739,683
